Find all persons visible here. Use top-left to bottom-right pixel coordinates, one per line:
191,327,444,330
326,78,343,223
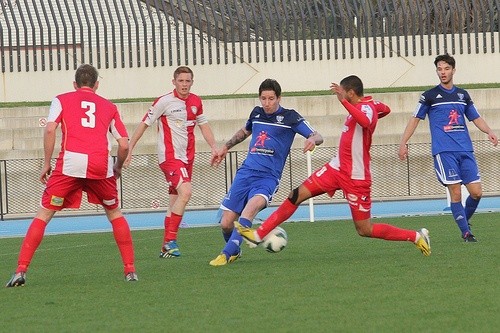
124,63,217,259
234,74,433,257
208,76,324,266
398,53,500,244
3,63,140,288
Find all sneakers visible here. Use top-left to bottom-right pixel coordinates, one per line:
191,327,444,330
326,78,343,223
234,220,265,248
462,229,477,242
160,249,177,258
209,247,242,265
126,271,139,280
6,271,26,287
164,239,181,255
416,226,431,256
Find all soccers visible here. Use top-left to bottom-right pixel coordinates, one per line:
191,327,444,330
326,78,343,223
262,226,287,254
